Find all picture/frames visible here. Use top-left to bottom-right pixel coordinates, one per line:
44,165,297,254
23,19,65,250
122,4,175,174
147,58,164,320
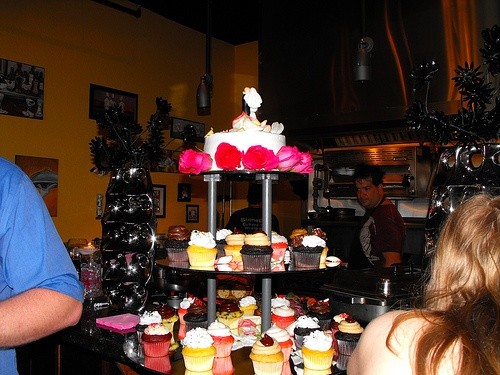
186,204,199,223
90,84,139,125
152,184,166,217
177,183,191,202
170,118,205,142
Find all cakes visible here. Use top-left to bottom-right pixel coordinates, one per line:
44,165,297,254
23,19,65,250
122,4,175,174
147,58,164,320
204,86,287,171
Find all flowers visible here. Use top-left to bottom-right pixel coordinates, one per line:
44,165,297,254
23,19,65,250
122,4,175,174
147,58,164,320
178,142,314,175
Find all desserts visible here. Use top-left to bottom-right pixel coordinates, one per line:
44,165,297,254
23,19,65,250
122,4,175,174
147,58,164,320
165,225,329,272
134,292,364,374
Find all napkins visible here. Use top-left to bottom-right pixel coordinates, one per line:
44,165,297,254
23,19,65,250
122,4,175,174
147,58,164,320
96,313,139,330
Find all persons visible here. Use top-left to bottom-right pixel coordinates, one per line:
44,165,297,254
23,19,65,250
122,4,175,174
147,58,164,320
354,164,407,268
224,189,280,235
0,157,85,375
347,196,500,375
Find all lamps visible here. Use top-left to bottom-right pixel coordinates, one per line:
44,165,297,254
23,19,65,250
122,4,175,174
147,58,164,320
353,37,373,81
197,73,212,108
